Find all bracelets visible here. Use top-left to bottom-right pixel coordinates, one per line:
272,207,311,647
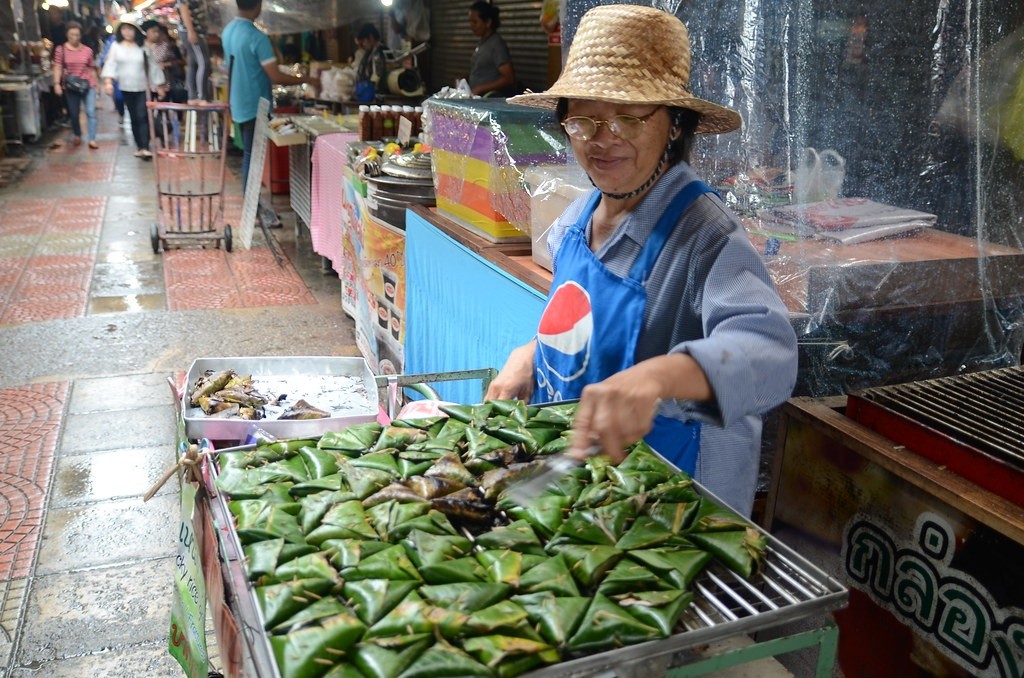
54,82,61,84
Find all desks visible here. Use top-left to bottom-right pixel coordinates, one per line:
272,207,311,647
212,81,367,274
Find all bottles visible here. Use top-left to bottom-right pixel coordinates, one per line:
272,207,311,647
358,104,423,141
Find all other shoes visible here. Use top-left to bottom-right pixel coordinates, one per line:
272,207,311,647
74,136,80,145
89,142,98,148
118,116,123,123
257,209,282,228
135,148,153,159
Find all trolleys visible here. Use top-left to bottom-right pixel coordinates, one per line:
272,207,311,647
137,48,236,251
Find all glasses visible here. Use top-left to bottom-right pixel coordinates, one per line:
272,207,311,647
560,103,663,142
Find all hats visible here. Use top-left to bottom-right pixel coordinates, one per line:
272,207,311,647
112,14,144,34
505,5,742,135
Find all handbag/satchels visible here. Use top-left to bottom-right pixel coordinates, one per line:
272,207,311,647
794,147,844,203
65,74,89,94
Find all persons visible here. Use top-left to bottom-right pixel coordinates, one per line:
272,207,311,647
347,24,393,79
142,20,186,151
102,17,168,157
99,33,126,123
54,21,99,148
220,0,320,228
486,3,797,522
175,0,222,106
459,0,514,95
